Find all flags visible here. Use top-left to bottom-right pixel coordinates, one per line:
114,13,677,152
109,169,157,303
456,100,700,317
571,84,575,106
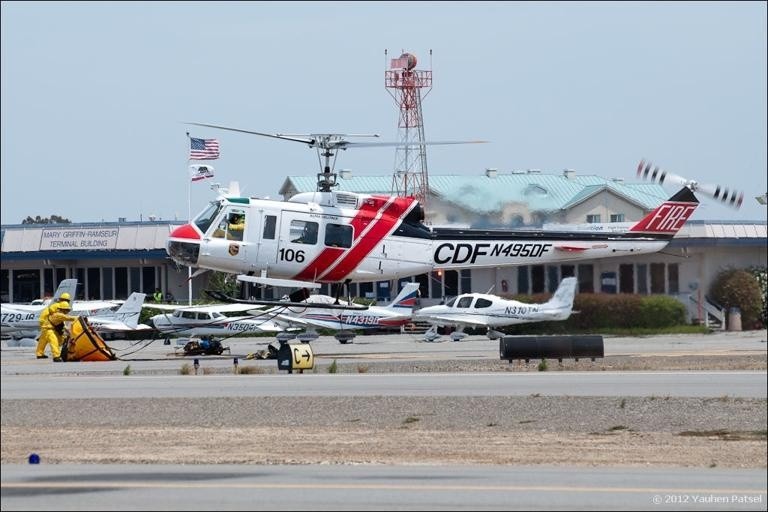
189,163,215,183
188,136,221,162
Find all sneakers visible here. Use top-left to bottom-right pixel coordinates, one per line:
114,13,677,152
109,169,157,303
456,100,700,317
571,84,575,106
53,356,62,362
37,355,49,359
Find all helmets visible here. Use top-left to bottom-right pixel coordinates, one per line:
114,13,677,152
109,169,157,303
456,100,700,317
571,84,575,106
57,301,72,310
59,292,72,301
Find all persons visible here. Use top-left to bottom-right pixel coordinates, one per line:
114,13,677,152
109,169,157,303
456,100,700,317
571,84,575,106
151,288,165,304
35,291,71,347
35,300,87,363
211,210,245,241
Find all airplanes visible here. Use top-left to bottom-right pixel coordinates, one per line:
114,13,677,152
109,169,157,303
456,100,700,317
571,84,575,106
2,277,152,348
147,301,295,346
412,275,582,343
265,281,422,343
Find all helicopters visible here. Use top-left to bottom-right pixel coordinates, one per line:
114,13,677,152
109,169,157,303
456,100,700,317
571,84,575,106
164,122,743,311
